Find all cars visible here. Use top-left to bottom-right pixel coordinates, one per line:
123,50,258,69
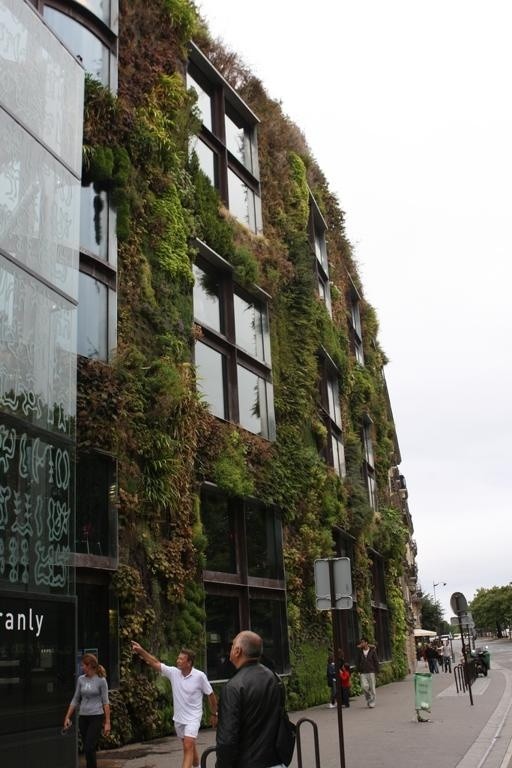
434,635,449,643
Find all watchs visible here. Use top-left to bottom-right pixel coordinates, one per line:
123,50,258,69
212,712,218,716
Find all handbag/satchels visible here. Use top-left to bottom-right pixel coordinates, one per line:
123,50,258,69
276,717,296,767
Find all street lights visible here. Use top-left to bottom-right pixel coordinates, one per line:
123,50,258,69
434,581,447,605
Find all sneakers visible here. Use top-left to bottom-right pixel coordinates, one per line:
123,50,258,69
369,703,375,707
326,704,334,708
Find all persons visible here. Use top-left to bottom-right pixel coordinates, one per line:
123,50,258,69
415,637,452,674
338,663,352,708
216,630,288,768
129,640,219,768
327,658,344,709
356,638,379,708
63,653,110,768
